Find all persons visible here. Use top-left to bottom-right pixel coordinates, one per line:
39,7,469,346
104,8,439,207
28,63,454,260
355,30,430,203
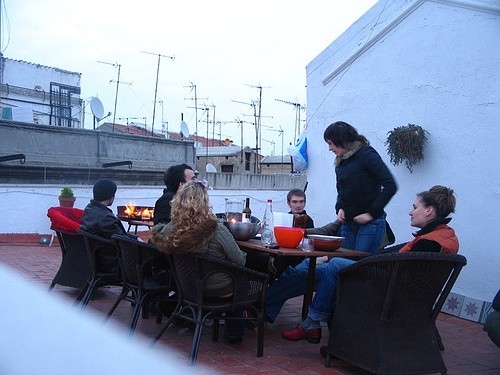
153,164,225,327
249,189,315,276
245,218,395,331
80,179,145,281
149,180,247,342
281,185,460,360
323,121,397,261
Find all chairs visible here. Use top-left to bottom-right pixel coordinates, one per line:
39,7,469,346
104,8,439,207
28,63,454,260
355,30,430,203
47,205,467,375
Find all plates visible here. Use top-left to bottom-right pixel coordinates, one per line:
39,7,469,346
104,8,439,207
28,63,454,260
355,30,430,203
307,235,345,239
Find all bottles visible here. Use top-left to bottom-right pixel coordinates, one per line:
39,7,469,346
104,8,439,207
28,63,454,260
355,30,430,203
261,200,274,245
242,198,252,223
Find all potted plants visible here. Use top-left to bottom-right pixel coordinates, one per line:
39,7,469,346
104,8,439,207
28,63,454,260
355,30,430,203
386,124,428,173
59,186,76,207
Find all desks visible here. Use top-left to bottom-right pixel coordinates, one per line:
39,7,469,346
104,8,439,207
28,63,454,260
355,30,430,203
127,220,372,322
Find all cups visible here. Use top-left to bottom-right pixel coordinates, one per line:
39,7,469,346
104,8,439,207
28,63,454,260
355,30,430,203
302,237,309,252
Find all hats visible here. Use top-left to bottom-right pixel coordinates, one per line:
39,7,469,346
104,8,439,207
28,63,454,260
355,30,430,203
94,179,117,200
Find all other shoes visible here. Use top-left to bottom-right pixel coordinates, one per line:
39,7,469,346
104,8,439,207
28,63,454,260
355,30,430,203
205,319,225,326
281,324,322,343
243,309,258,328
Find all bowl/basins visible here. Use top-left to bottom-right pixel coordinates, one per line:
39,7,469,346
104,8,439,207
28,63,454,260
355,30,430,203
310,238,342,252
274,227,305,248
223,222,261,241
